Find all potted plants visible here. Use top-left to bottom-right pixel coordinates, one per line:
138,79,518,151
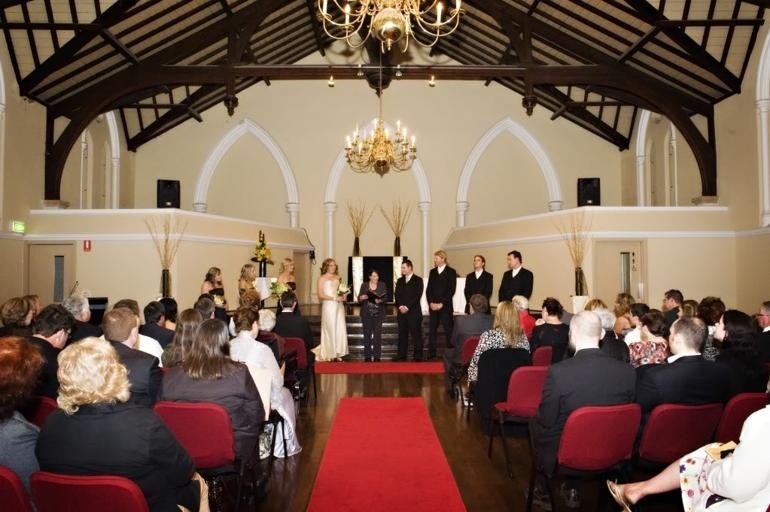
148,215,187,297
346,200,375,256
555,211,593,296
380,202,412,256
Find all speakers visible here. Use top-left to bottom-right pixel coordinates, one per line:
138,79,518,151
577,177,602,206
156,178,180,207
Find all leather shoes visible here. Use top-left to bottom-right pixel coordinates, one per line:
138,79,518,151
560,482,581,508
364,357,380,362
525,487,550,510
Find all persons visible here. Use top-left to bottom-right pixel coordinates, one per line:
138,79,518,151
0,287,302,508
447,290,769,510
463,256,493,315
356,271,388,361
315,258,348,362
236,263,257,306
424,251,457,361
391,260,423,362
274,257,300,317
200,266,228,322
498,250,533,306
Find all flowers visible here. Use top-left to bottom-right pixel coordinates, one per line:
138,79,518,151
212,294,226,306
336,282,348,295
253,229,272,262
269,280,291,298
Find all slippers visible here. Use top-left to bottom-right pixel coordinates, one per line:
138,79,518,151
606,479,632,511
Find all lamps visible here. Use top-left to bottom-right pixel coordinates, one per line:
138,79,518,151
342,64,418,177
313,0,463,56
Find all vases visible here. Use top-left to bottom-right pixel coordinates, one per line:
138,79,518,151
259,258,267,278
